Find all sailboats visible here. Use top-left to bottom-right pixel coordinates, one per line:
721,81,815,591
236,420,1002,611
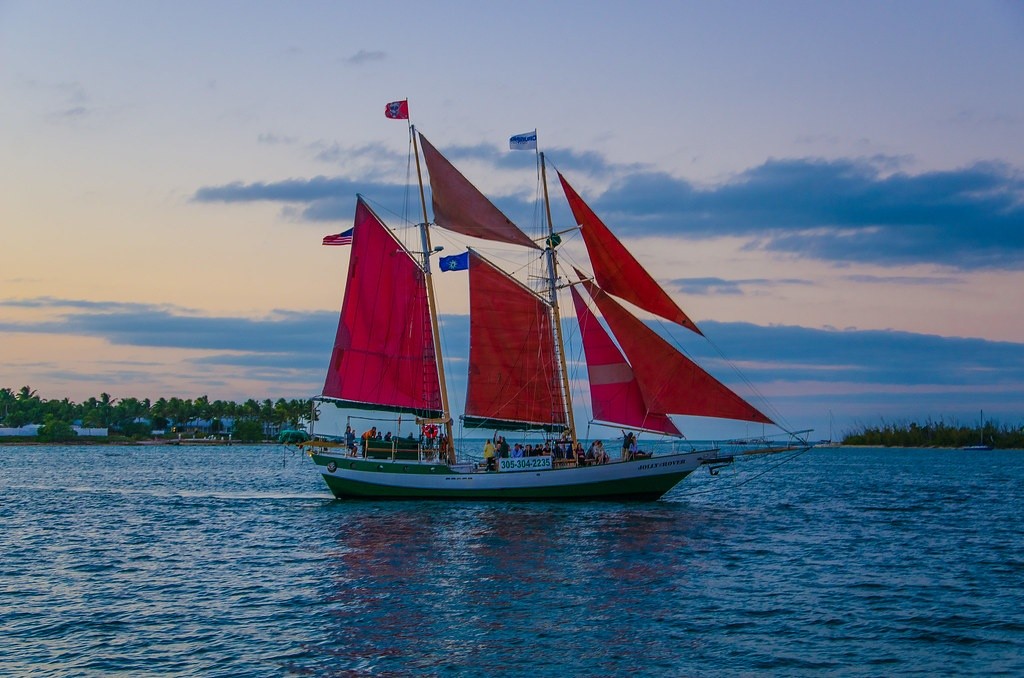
310,98,814,504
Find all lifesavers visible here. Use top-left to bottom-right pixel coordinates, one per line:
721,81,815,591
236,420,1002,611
425,425,438,438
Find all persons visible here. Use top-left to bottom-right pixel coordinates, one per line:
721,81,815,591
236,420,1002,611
343,426,358,457
406,432,449,460
361,427,393,458
483,432,609,471
178,432,231,441
621,429,638,462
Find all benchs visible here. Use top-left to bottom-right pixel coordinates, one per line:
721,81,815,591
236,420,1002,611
366,440,419,459
586,458,601,465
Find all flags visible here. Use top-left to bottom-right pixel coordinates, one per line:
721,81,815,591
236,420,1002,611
439,251,470,272
385,100,409,120
509,129,537,151
322,226,354,246
